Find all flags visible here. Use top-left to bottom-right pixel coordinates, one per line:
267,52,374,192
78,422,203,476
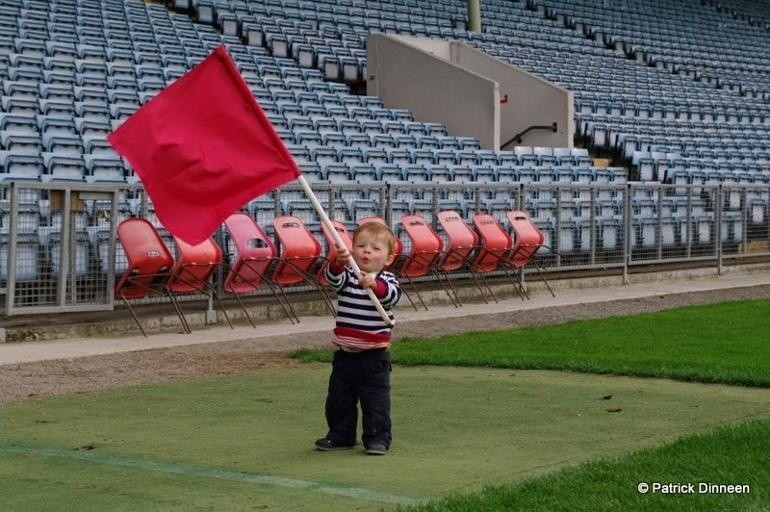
108,44,302,246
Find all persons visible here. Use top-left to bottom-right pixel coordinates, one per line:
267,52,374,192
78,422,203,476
316,222,401,455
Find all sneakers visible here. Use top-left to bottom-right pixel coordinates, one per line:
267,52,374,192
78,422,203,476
315,436,356,450
364,441,387,455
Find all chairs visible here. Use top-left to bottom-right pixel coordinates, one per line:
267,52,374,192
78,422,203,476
0,0,770,338
